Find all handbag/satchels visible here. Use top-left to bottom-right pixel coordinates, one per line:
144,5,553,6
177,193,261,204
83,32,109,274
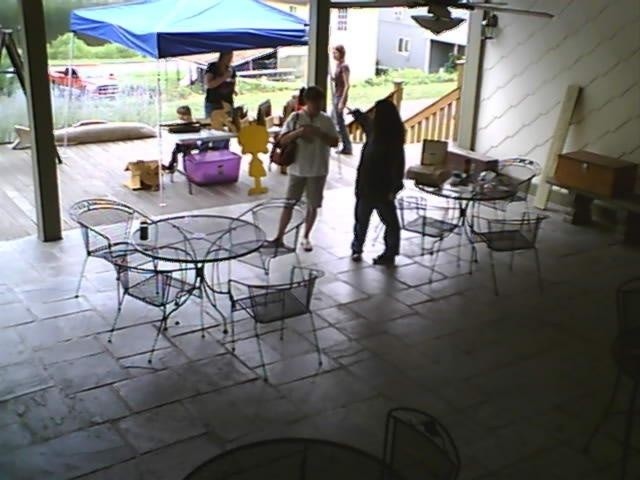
269,134,299,165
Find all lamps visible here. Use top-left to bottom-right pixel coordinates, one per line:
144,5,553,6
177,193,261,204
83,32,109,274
412,8,466,34
481,14,499,38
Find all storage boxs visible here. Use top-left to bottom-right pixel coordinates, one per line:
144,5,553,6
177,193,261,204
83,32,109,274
553,150,638,198
184,150,242,185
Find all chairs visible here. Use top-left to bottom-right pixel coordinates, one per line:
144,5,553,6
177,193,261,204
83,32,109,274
397,197,465,283
212,198,307,305
229,266,323,381
382,406,462,479
470,158,542,234
582,277,639,478
469,211,551,296
68,198,159,311
103,247,205,362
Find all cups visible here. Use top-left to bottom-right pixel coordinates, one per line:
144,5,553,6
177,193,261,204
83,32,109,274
140,221,148,240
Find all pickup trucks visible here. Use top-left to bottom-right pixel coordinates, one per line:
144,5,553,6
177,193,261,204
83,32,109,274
49,64,122,101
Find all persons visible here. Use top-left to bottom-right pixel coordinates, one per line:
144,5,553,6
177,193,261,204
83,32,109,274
162,106,202,174
203,50,237,149
330,46,353,155
270,86,339,251
346,99,405,264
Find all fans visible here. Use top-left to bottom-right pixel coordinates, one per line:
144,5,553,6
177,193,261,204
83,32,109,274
329,1,556,20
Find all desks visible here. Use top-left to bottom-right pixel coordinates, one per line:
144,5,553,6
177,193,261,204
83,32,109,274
181,438,405,480
160,118,290,195
131,214,266,339
547,176,640,243
415,172,518,261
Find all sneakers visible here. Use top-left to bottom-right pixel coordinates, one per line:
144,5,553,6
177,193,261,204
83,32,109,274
336,148,352,155
352,249,362,262
301,239,312,251
373,253,394,265
